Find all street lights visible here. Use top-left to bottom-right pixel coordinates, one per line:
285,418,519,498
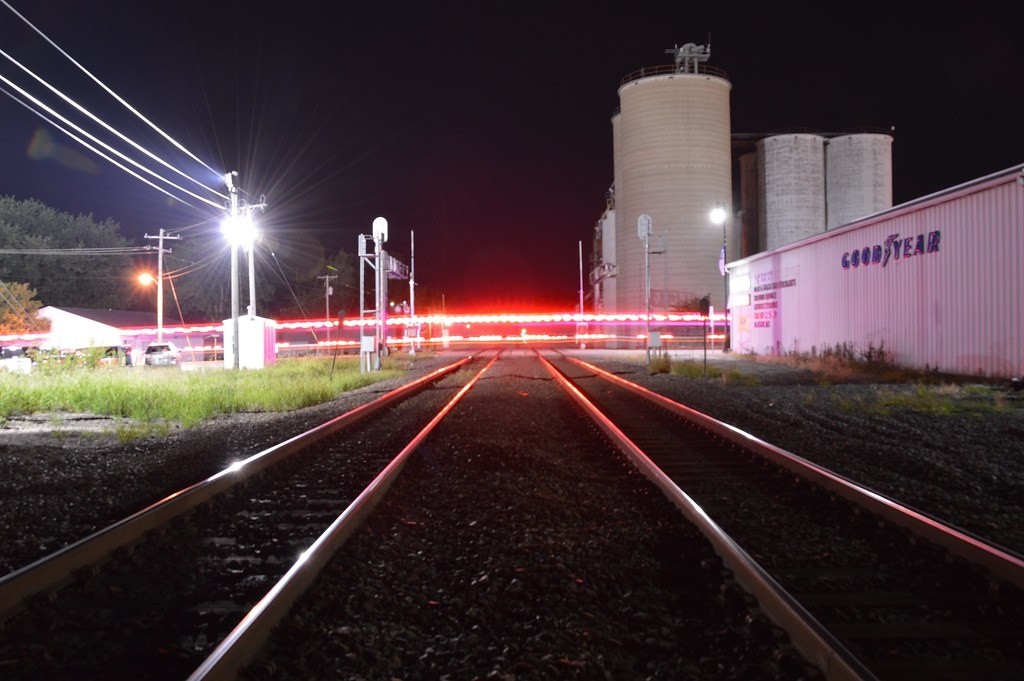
135,274,163,344
710,204,729,338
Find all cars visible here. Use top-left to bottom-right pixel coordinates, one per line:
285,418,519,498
143,341,183,368
